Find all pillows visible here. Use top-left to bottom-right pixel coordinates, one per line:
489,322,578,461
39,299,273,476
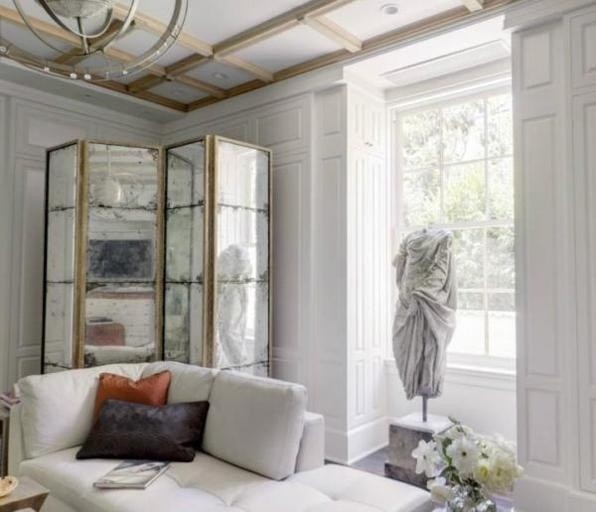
76,372,211,463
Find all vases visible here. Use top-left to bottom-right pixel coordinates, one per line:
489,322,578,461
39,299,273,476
445,482,496,510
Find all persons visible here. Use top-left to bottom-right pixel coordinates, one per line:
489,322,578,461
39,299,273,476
101,461,165,484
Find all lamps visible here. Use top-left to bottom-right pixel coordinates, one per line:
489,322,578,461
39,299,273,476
0,1,188,80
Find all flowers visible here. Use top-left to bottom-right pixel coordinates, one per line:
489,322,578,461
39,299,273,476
410,415,522,509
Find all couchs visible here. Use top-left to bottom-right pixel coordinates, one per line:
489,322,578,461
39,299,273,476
7,362,433,509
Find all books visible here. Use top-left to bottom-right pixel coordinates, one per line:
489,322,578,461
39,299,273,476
93,459,172,489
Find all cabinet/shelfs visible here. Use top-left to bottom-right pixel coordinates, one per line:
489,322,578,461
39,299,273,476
39,135,272,374
